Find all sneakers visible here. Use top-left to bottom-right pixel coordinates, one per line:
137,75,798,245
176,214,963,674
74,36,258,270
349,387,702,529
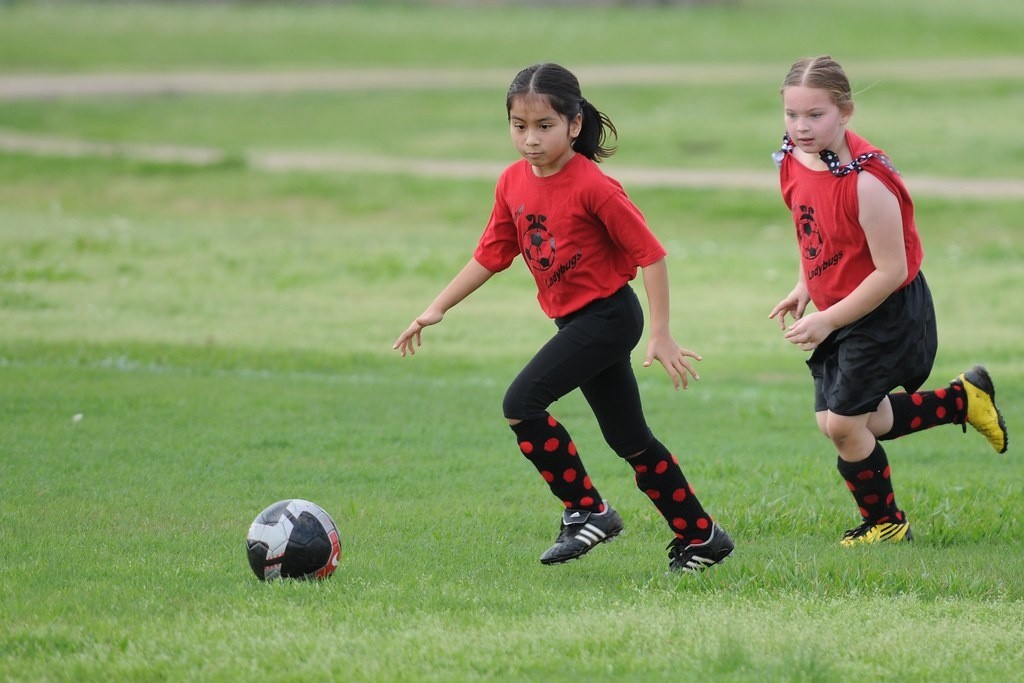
664,523,736,581
541,500,624,565
839,510,913,546
949,367,1008,455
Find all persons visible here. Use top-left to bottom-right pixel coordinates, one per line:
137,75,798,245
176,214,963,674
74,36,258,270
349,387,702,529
393,62,735,579
768,55,1010,547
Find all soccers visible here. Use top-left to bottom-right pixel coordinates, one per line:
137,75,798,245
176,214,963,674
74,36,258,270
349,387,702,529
246,498,342,583
